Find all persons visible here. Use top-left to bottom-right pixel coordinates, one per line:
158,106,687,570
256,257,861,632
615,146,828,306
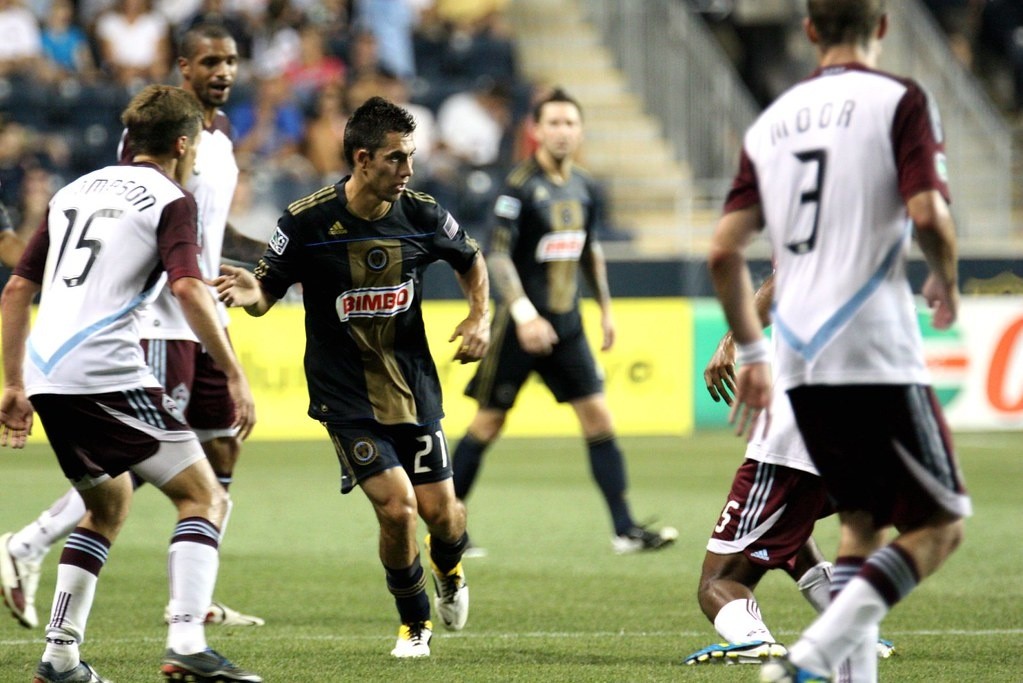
0,22,270,632
213,91,497,660
1,84,268,681
680,267,899,667
705,0,982,683
424,86,680,558
0,0,564,295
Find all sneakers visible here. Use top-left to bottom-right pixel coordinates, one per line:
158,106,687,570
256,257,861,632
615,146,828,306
391,619,435,659
876,639,896,659
165,600,265,627
161,647,264,683
36,659,114,683
683,642,790,665
756,656,835,683
424,532,469,634
423,534,485,560
1,532,41,630
611,526,678,554
